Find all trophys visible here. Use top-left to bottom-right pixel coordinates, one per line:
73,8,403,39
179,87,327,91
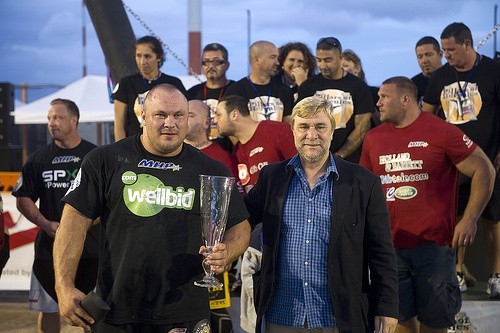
194,174,235,287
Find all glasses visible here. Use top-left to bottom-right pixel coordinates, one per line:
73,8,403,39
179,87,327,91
316,38,341,53
202,60,226,66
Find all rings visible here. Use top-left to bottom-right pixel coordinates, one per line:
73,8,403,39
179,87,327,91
464,240,469,243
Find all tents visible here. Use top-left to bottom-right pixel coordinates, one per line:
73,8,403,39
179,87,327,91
12,73,207,169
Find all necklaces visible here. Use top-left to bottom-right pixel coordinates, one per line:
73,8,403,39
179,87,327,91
341,67,345,78
148,73,160,84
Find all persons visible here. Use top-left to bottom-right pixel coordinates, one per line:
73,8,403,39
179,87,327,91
0,23,500,333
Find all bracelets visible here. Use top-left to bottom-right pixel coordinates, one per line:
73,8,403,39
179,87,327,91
334,152,343,158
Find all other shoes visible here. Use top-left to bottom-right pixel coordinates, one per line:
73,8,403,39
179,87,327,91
486,276,500,296
456,275,467,292
